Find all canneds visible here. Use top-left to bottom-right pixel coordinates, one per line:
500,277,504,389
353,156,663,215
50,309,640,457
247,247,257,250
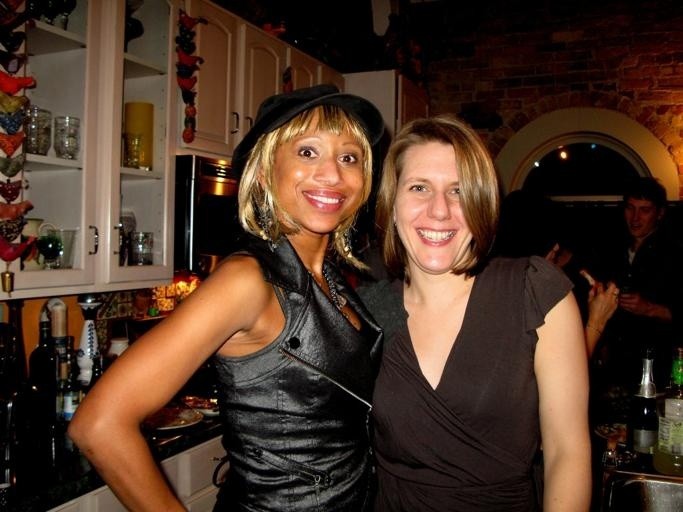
129,232,154,266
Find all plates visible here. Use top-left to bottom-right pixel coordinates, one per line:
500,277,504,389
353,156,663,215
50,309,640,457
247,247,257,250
595,422,627,447
196,397,222,417
153,408,205,431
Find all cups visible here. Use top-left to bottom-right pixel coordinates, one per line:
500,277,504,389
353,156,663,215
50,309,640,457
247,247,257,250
22,103,82,159
119,209,154,265
19,218,79,270
651,415,683,478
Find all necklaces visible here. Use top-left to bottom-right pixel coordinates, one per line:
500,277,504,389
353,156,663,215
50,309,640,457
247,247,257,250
307,268,349,320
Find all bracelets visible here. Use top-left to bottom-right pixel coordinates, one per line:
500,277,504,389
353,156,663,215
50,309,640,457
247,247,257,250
586,324,601,337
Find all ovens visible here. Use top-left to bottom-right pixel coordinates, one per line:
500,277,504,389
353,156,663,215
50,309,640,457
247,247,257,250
172,152,244,277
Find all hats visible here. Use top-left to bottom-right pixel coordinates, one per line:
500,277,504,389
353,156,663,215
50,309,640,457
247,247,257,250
230,82,384,180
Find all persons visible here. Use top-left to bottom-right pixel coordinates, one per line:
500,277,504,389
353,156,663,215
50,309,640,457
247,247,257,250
65,85,384,512
352,115,596,511
618,176,683,403
504,189,620,360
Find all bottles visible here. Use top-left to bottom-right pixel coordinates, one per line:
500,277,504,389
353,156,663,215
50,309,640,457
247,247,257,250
0,299,129,490
627,347,660,467
665,345,683,417
